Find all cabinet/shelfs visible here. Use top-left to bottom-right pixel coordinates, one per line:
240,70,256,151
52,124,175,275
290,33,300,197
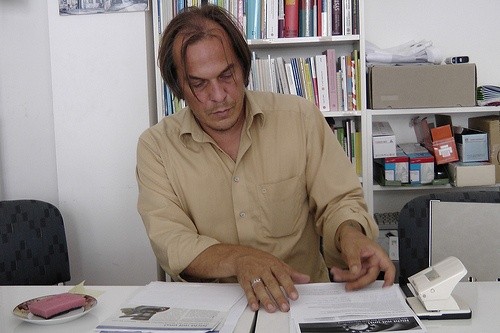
374,103,500,192
151,0,375,221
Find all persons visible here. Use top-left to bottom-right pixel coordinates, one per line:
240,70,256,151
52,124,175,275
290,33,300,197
136,3,396,313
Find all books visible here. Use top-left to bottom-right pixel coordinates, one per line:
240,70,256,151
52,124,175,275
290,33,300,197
162,49,361,117
331,119,362,177
153,0,360,41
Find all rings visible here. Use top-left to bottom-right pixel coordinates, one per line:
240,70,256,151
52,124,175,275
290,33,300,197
251,278,262,286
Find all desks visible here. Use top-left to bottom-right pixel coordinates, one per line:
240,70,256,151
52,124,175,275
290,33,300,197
0,279,257,333
255,283,500,333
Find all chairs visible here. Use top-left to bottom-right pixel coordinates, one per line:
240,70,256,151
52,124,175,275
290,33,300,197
397,191,500,282
0,199,71,287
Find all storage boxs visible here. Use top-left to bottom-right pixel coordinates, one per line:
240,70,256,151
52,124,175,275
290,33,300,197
368,62,481,110
376,114,500,187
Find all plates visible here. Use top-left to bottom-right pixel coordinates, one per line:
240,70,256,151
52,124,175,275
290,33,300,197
13,292,98,326
